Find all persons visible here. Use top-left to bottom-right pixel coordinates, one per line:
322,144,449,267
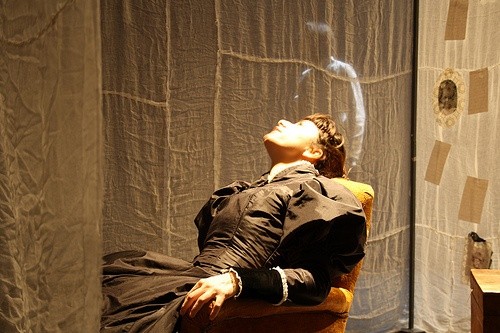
95,110,368,333
294,19,365,180
439,86,457,114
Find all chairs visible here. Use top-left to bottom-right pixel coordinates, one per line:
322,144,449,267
179,178,374,333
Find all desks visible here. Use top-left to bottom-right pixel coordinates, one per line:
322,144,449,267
469,266,500,333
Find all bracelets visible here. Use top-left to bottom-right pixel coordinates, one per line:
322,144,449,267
229,273,235,298
